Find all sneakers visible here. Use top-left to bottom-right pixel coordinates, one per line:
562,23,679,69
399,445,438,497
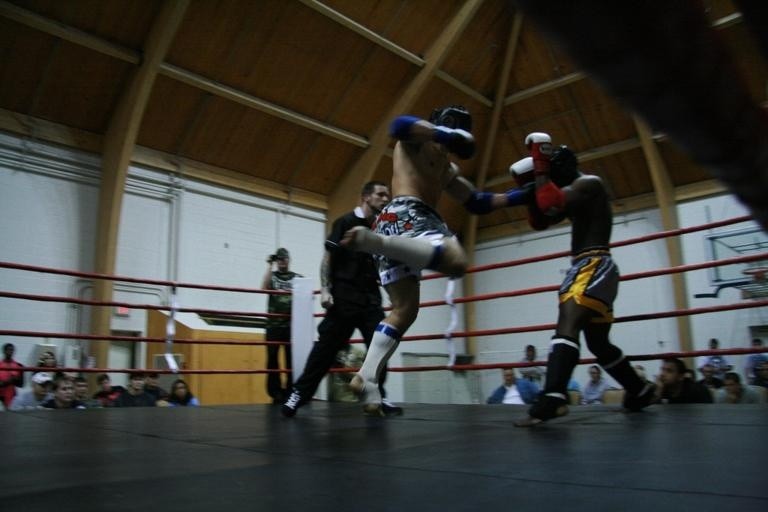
281,389,308,418
382,402,402,418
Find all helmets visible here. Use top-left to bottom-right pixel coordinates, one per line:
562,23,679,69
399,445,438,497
428,103,473,136
548,143,579,188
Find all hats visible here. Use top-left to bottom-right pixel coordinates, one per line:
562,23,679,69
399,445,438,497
31,372,52,385
271,248,289,260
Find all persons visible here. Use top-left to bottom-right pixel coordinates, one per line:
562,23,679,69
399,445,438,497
509,132,657,428
0,344,199,414
339,104,532,418
262,248,302,403
487,337,768,405
282,180,402,417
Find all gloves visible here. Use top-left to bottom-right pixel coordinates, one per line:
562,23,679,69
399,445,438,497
432,124,477,159
523,130,552,178
509,155,536,187
507,180,536,208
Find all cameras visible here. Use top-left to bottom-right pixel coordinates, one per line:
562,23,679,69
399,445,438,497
267,255,284,264
